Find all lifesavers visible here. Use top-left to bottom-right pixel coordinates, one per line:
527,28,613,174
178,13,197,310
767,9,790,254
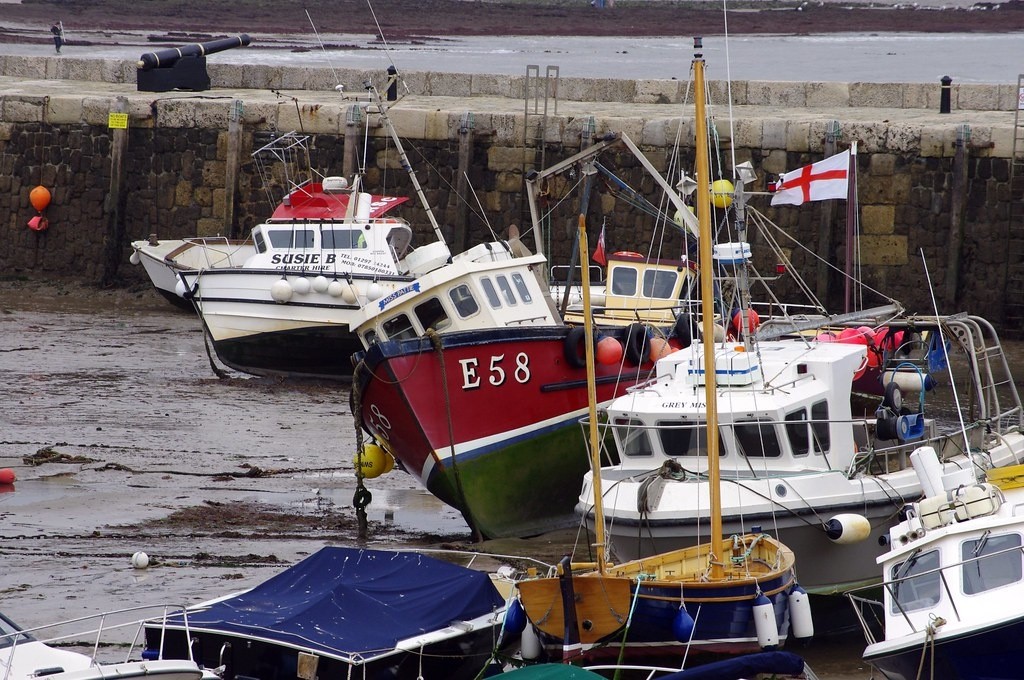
613,250,645,259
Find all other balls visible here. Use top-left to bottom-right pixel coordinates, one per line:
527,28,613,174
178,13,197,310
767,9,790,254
29,185,51,212
294,277,311,294
365,283,383,301
352,443,394,479
312,275,329,292
129,253,141,265
596,336,623,366
731,308,760,333
270,279,293,302
132,551,149,568
327,280,343,296
0,468,16,484
648,337,672,364
175,280,188,298
342,285,360,305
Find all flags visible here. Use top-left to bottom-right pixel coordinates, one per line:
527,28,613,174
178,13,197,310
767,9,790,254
591,226,606,266
768,149,850,205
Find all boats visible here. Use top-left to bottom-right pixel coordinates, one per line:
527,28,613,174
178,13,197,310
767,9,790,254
0,50,1024,680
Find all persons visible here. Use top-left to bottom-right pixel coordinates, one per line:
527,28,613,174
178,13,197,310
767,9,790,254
51,23,62,54
